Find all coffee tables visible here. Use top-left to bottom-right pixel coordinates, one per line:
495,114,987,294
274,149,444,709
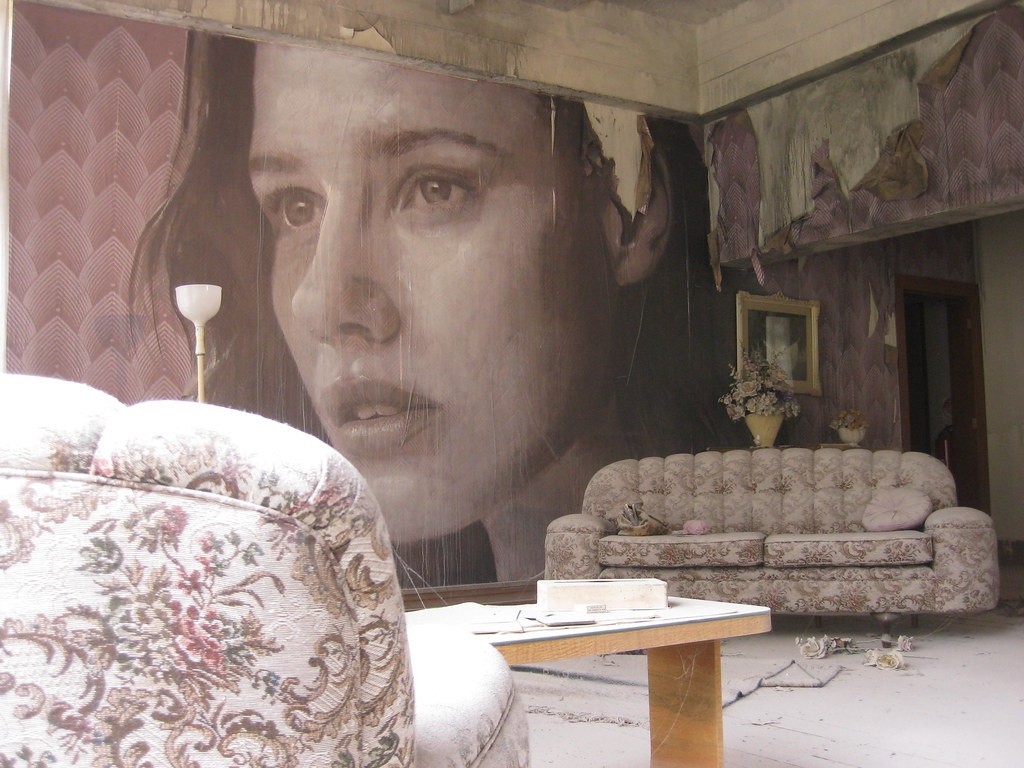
437,592,772,767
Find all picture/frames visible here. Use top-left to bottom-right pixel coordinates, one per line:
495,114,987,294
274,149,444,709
731,293,824,396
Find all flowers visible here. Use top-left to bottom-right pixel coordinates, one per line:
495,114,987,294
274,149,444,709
830,411,871,432
717,343,800,422
795,615,957,669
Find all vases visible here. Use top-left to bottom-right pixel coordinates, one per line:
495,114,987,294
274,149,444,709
840,428,866,446
746,412,783,447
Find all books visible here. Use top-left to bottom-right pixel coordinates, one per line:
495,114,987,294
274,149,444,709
464,601,596,635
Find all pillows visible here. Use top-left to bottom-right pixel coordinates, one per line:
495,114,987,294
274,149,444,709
860,488,932,530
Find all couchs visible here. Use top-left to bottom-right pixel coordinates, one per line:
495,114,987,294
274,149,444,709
0,371,530,768
543,446,995,648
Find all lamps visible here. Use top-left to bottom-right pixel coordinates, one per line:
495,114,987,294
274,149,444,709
175,284,223,402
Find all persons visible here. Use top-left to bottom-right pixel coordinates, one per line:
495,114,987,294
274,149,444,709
126,31,795,588
